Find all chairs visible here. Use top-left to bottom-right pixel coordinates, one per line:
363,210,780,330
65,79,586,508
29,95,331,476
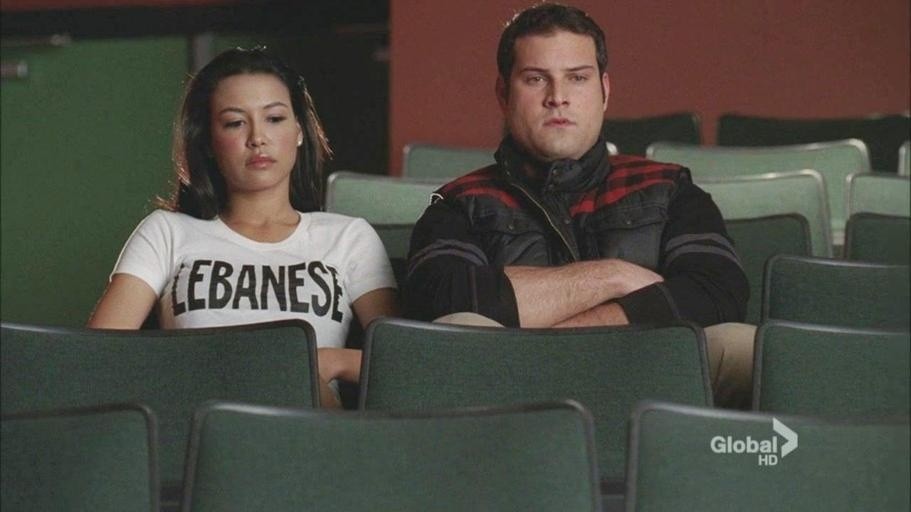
1,111,911,512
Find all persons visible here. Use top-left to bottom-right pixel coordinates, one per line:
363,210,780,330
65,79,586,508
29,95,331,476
88,48,403,419
405,2,759,410
